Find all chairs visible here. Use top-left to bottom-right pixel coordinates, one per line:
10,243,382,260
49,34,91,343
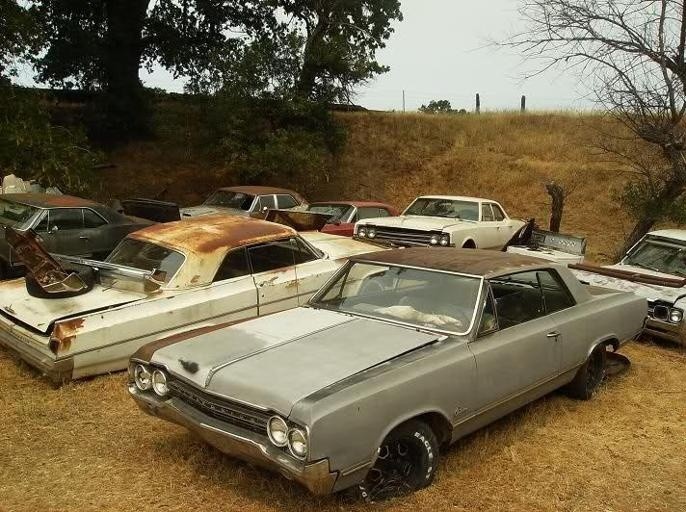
464,294,501,336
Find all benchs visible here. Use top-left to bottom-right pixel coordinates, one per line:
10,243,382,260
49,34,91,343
483,283,568,324
